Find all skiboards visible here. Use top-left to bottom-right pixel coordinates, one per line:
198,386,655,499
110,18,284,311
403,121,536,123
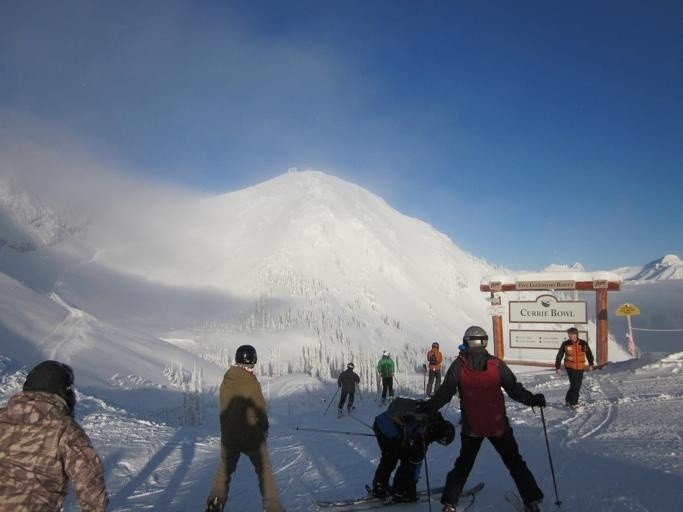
336,406,356,418
321,480,485,511
444,489,542,512
379,397,394,407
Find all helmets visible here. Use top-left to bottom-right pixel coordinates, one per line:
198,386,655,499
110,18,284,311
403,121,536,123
432,343,439,348
383,351,390,357
347,362,354,368
236,344,256,364
463,326,488,347
23,361,76,409
436,420,455,447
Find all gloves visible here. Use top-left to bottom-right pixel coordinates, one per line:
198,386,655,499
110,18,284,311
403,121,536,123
530,393,546,408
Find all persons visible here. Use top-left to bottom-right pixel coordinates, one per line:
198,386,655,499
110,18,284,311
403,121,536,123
416,326,546,512
337,363,360,416
426,342,442,398
378,351,396,401
373,396,455,503
206,345,285,512
0,360,110,512
555,327,594,410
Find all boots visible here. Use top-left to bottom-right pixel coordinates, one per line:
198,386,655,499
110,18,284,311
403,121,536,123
372,480,416,504
523,501,540,512
442,503,456,512
337,407,352,418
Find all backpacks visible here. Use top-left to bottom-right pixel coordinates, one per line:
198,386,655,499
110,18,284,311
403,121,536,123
430,354,437,365
